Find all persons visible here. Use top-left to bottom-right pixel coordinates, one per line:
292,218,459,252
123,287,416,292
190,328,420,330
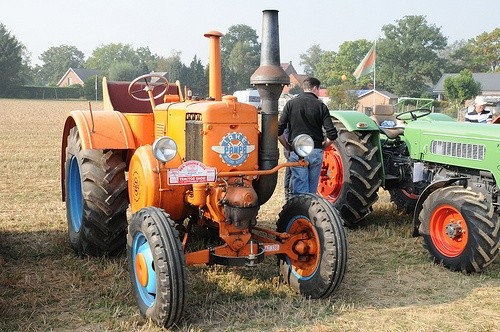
276,79,339,203
463,94,493,124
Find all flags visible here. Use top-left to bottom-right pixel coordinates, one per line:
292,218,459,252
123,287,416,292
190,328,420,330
353,40,377,81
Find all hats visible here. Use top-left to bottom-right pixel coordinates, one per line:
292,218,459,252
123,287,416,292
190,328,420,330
475,96,486,106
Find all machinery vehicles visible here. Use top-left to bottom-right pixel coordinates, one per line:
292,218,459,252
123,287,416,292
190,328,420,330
317,105,500,274
60,9,347,329
